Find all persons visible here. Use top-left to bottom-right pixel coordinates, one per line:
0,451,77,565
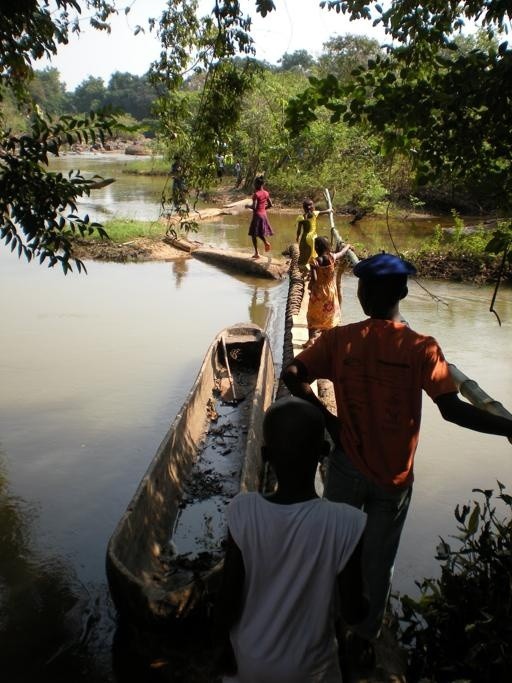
304,235,352,348
234,160,245,189
282,251,511,679
216,152,226,181
294,197,334,267
209,397,370,681
245,174,274,258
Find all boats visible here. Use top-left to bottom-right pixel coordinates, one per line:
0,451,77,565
105,321,276,644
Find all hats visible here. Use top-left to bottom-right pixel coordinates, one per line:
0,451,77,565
353,250,417,281
